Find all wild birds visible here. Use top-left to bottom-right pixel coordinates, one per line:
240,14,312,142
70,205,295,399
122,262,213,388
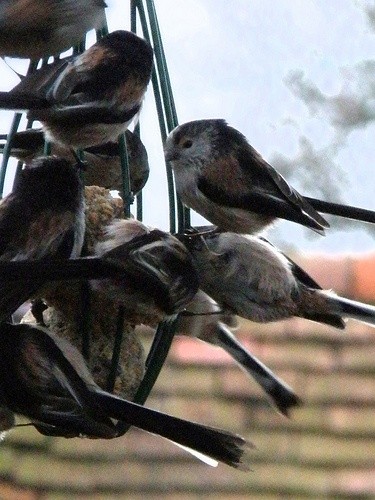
20,296,146,399
164,118,331,244
0,315,256,473
1,155,99,319
0,217,202,321
90,259,301,419
0,126,149,193
172,222,351,330
107,0,157,58
